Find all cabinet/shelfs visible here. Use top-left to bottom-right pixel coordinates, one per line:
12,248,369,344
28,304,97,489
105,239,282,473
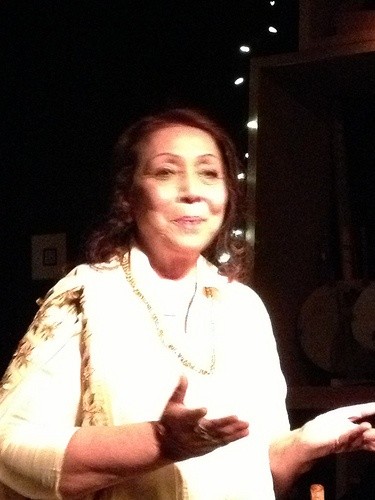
244,42,375,411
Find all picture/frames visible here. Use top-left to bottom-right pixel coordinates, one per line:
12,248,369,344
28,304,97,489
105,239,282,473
27,227,74,288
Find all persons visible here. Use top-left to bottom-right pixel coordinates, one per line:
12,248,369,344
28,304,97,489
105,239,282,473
0,105,375,500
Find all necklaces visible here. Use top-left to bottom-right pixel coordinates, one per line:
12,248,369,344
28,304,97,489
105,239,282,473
117,251,218,377
